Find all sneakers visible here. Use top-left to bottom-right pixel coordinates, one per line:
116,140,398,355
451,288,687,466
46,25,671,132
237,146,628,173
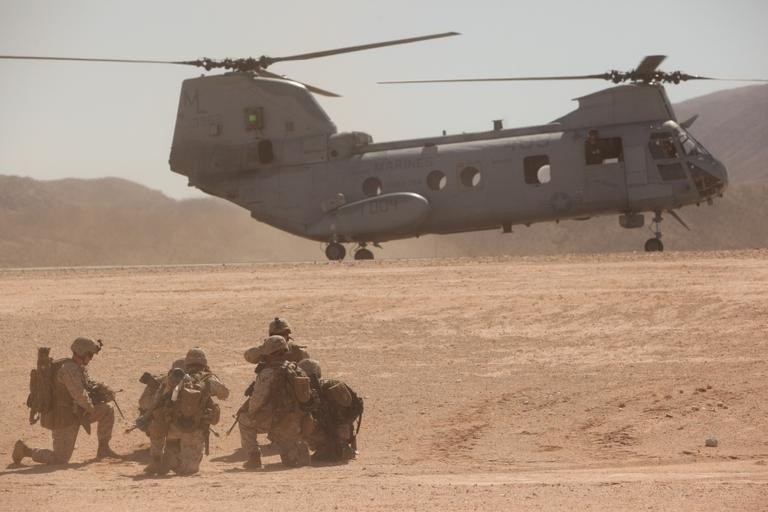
94,447,125,459
292,438,333,467
143,456,171,475
11,438,32,467
241,447,264,471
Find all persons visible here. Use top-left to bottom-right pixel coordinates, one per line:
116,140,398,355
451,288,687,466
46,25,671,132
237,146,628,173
236,334,315,471
11,335,124,466
137,355,188,461
242,316,311,368
585,134,612,164
295,357,364,463
660,141,676,160
148,346,230,475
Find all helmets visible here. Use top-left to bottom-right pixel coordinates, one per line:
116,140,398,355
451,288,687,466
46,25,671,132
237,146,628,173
295,357,322,379
71,337,101,357
259,334,291,357
170,346,210,379
267,316,292,338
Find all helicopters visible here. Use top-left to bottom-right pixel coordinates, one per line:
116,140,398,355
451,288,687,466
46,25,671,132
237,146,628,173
0,32,768,261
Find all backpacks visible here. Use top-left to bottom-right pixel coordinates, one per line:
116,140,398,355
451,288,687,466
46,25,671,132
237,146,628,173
318,377,365,425
135,376,173,412
168,371,218,427
25,356,71,414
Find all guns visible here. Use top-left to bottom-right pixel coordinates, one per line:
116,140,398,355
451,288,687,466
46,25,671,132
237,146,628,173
139,372,219,437
226,381,255,436
